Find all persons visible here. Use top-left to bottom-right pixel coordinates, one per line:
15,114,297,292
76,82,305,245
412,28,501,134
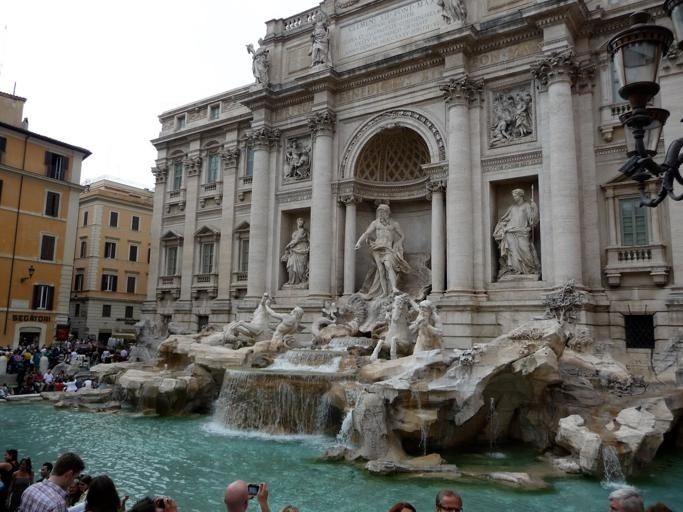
291,143,311,179
0,332,130,396
353,203,405,297
488,90,532,146
607,488,644,512
389,502,415,512
492,187,539,278
284,138,299,181
435,489,461,512
219,288,447,363
281,216,308,286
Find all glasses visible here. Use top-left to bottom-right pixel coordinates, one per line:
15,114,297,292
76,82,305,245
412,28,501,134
20,264,35,283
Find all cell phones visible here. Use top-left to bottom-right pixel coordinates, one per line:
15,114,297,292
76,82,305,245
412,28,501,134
247,484,259,496
